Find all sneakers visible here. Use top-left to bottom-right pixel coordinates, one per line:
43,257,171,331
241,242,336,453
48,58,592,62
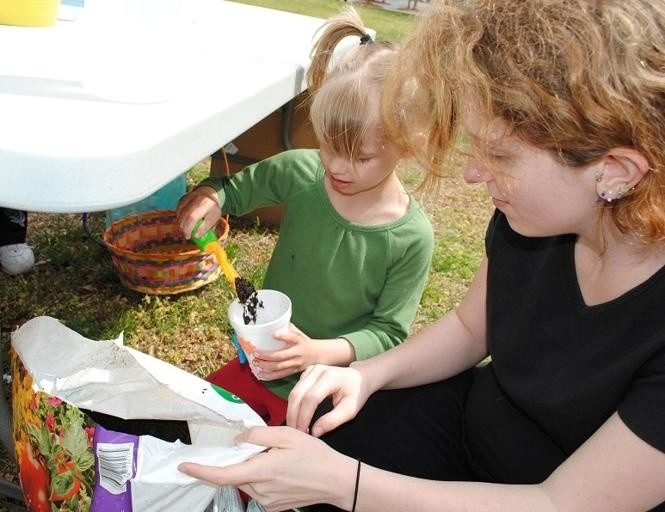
0,242,36,277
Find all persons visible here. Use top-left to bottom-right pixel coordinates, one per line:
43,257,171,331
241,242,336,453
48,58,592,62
176,5,435,427
0,206,35,276
176,0,664,512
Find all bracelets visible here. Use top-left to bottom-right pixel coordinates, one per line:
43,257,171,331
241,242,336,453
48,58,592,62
351,460,361,512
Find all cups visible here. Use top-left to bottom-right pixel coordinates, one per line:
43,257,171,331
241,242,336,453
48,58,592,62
227,288,292,382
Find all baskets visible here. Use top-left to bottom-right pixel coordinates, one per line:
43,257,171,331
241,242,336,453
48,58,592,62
103,208,231,296
